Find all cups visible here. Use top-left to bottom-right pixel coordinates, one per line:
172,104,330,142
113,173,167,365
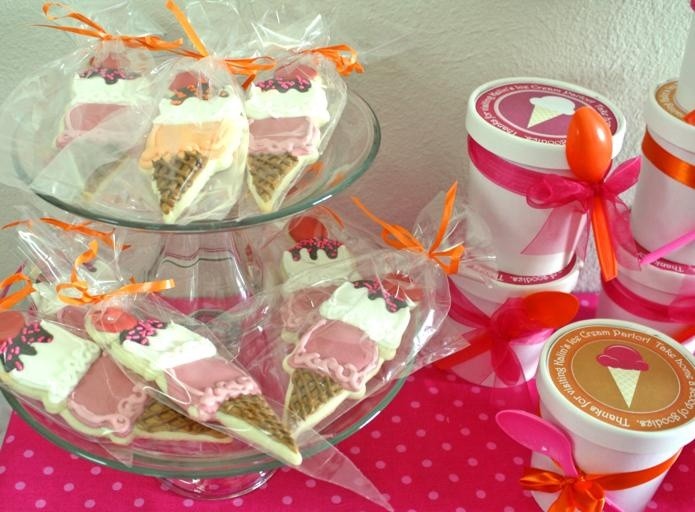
672,1,694,113
596,207,693,338
461,78,626,279
534,317,695,512
429,220,581,396
630,78,694,272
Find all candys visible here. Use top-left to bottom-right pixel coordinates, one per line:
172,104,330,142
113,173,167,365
0,237,415,465
48,58,330,226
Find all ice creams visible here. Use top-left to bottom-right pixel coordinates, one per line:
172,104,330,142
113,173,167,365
596,344,650,409
527,96,576,128
